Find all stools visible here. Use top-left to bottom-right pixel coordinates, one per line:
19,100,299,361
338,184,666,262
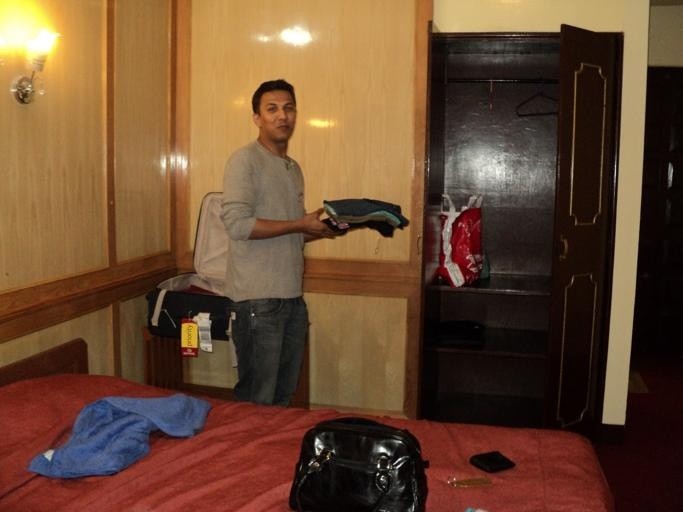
141,321,314,410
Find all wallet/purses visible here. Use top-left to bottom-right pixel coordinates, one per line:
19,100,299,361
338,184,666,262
470,451,514,473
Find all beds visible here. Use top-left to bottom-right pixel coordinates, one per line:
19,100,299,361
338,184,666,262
2,335,617,512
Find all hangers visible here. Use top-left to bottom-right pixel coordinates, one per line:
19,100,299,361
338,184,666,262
515,78,559,118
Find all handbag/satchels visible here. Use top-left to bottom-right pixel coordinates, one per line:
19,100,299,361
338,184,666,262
289,417,428,512
439,194,489,288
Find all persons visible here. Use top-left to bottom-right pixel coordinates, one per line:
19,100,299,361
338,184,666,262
219,78,340,407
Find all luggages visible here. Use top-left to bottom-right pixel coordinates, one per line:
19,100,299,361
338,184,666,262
146,193,231,341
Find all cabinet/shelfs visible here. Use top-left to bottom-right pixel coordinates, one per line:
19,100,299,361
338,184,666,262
416,14,626,445
